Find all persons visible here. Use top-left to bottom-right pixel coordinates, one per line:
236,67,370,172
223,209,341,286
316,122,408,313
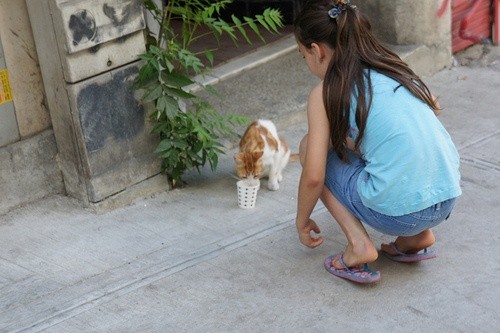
296,0,462,283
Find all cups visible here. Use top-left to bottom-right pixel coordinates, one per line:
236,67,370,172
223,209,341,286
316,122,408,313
237,180,259,209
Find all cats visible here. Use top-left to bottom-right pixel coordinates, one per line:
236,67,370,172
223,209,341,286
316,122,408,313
233,119,291,191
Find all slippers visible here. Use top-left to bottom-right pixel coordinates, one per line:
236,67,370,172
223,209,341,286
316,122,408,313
325,255,381,283
381,242,437,261
